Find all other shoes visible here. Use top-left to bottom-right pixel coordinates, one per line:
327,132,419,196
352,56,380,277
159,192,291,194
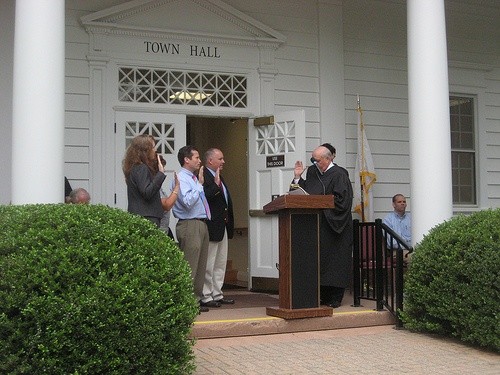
197,306,209,315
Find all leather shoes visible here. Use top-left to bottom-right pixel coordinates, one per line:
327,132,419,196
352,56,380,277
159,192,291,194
199,297,234,308
329,302,340,307
320,299,325,305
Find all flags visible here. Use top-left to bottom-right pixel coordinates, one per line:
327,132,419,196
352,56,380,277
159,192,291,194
351,107,377,222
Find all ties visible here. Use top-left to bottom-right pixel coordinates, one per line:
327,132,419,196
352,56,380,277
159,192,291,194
192,175,212,220
214,173,228,222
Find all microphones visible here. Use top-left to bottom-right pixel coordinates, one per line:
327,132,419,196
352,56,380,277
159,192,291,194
311,158,323,176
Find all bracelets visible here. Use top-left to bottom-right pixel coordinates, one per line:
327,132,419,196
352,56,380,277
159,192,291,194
171,190,178,196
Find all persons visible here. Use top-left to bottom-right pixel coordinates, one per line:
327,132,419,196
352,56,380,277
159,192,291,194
121,135,179,229
199,147,236,306
291,142,353,308
381,195,413,263
64,177,90,203
170,146,212,315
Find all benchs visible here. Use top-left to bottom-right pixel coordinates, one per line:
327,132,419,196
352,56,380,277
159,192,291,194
357,221,411,292
